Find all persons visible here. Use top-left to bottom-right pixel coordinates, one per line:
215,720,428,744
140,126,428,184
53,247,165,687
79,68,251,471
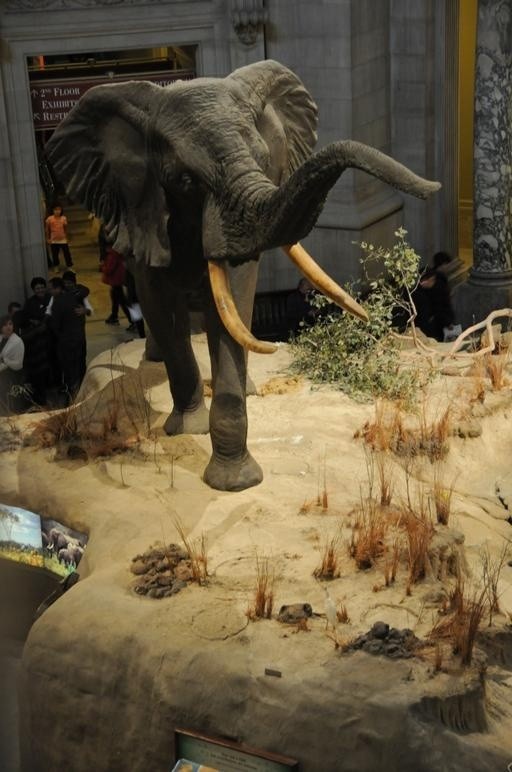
121,259,147,339
432,250,462,345
287,275,327,339
400,264,441,336
44,200,75,273
1,265,99,415
99,241,139,330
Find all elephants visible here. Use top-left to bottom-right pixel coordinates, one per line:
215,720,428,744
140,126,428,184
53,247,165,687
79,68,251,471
42,58,444,492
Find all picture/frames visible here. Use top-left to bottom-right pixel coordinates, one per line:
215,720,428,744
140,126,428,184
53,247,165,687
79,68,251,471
173,728,299,772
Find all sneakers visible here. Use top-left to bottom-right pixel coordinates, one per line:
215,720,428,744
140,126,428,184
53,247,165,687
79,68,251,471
125,325,138,333
104,318,120,325
